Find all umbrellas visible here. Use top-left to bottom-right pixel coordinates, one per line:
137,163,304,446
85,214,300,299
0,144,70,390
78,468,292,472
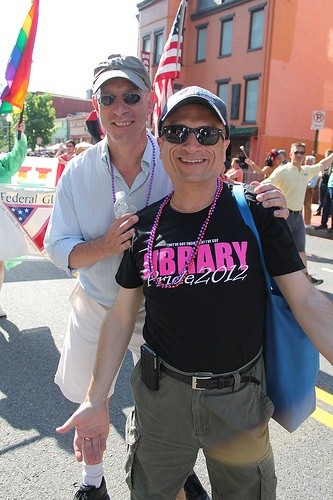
27,140,94,152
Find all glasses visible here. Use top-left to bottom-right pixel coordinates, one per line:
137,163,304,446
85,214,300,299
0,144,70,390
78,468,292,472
96,92,145,107
292,150,306,155
159,124,224,146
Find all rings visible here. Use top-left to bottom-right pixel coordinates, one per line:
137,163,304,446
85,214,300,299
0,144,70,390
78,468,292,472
84,438,91,440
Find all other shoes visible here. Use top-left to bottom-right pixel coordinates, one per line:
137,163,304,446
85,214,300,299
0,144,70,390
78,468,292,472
0,306,6,317
315,224,327,230
306,275,323,285
327,228,333,233
303,224,311,229
73,476,110,500
183,472,210,500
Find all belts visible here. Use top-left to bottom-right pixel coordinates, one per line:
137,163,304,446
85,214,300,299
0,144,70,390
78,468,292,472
159,352,264,391
288,209,302,215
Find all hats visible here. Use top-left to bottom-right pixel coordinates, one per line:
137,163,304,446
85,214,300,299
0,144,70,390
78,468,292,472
159,85,230,139
93,53,151,98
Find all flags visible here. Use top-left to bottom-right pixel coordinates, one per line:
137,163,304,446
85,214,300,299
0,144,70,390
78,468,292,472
0,0,40,115
152,0,188,137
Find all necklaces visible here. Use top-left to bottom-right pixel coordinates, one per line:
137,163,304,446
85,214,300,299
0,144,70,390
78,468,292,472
147,177,220,287
110,134,155,207
150,178,223,288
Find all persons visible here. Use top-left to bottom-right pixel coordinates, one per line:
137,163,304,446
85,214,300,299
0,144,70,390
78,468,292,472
0,119,28,317
55,86,333,500
54,140,76,187
224,142,333,287
27,152,49,157
43,55,288,500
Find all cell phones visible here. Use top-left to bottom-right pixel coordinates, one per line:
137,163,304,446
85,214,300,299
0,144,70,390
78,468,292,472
139,345,158,391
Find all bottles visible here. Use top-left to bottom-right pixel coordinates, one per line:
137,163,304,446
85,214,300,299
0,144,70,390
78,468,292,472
113,190,138,220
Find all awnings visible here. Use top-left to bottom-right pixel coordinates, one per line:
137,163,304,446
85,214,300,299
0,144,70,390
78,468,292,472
230,127,257,136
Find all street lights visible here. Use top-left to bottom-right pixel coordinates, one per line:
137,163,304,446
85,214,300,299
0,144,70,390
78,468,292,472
6,112,13,152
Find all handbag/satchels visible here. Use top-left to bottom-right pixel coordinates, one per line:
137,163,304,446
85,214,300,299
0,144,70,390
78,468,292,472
232,185,320,433
